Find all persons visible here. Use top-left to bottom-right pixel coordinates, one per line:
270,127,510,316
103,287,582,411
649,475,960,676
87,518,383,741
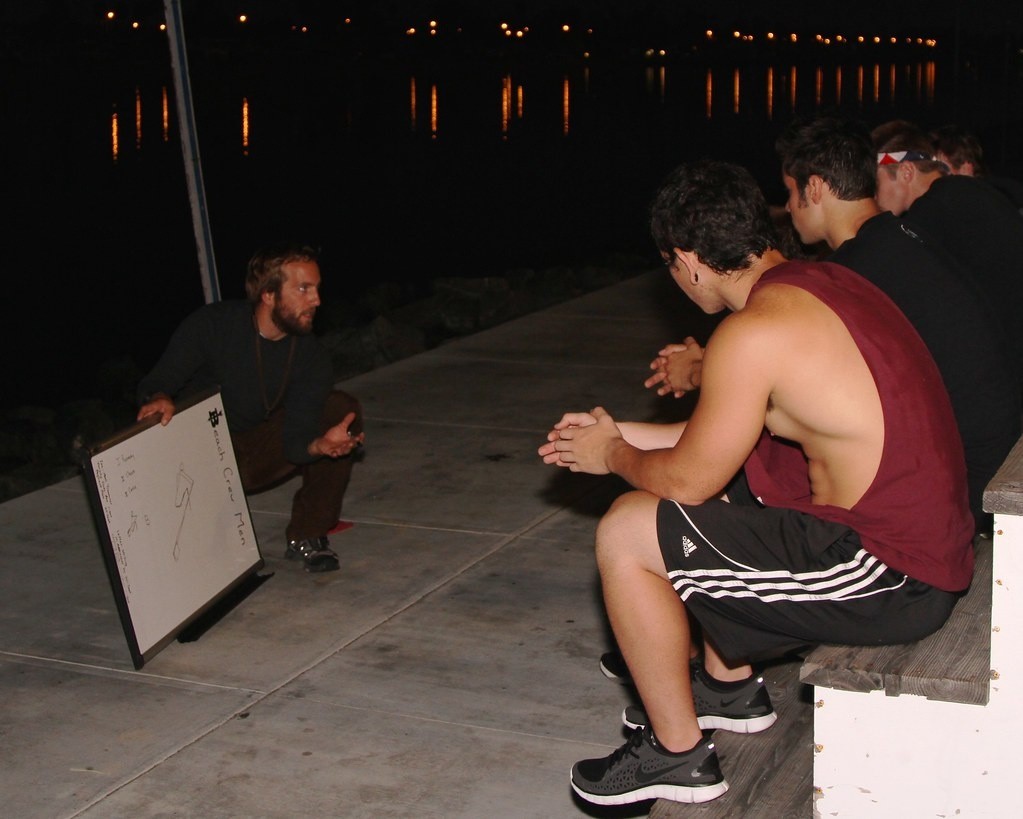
860,116,1021,334
134,242,369,572
598,111,1010,685
536,157,974,807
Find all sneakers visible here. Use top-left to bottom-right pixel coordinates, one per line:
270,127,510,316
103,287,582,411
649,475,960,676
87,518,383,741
599,648,706,684
621,665,778,733
570,725,730,806
285,535,340,573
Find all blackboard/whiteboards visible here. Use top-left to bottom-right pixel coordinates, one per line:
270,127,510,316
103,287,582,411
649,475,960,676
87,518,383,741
82,385,267,671
